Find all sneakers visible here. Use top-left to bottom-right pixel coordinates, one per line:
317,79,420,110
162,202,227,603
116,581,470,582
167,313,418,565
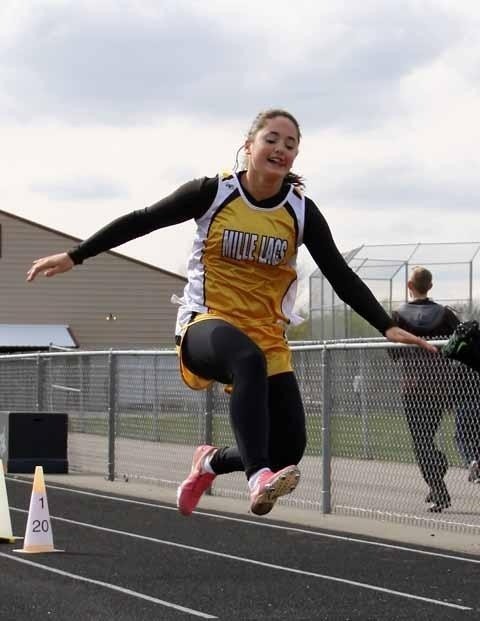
424,491,451,511
248,465,300,516
468,461,480,482
176,445,218,516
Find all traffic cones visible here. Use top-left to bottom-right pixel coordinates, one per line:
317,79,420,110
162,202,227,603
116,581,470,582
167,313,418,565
12,466,65,554
0,459,16,544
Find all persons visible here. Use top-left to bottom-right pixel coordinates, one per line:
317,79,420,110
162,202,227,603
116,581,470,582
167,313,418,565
386,265,461,514
453,362,480,485
26,108,439,516
